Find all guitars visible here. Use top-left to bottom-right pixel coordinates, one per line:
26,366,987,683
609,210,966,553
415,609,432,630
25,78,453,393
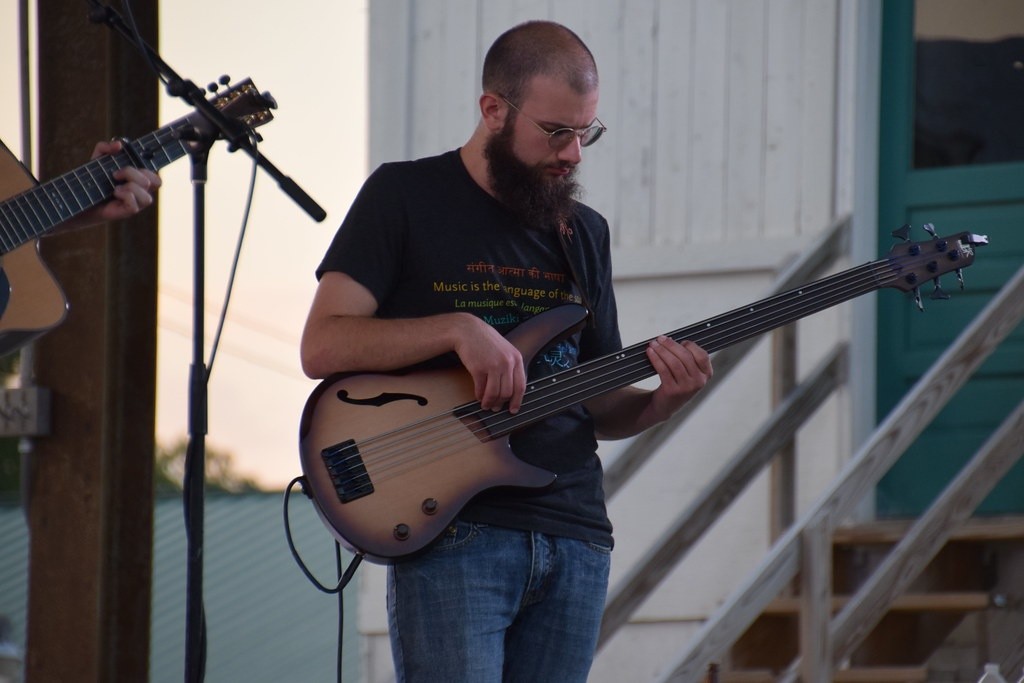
0,73,278,363
298,224,990,569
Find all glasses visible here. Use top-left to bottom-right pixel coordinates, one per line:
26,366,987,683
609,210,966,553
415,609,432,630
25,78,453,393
499,95,607,150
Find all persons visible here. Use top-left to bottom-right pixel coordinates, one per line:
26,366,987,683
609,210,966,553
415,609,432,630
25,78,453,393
37,138,163,234
298,19,716,682
